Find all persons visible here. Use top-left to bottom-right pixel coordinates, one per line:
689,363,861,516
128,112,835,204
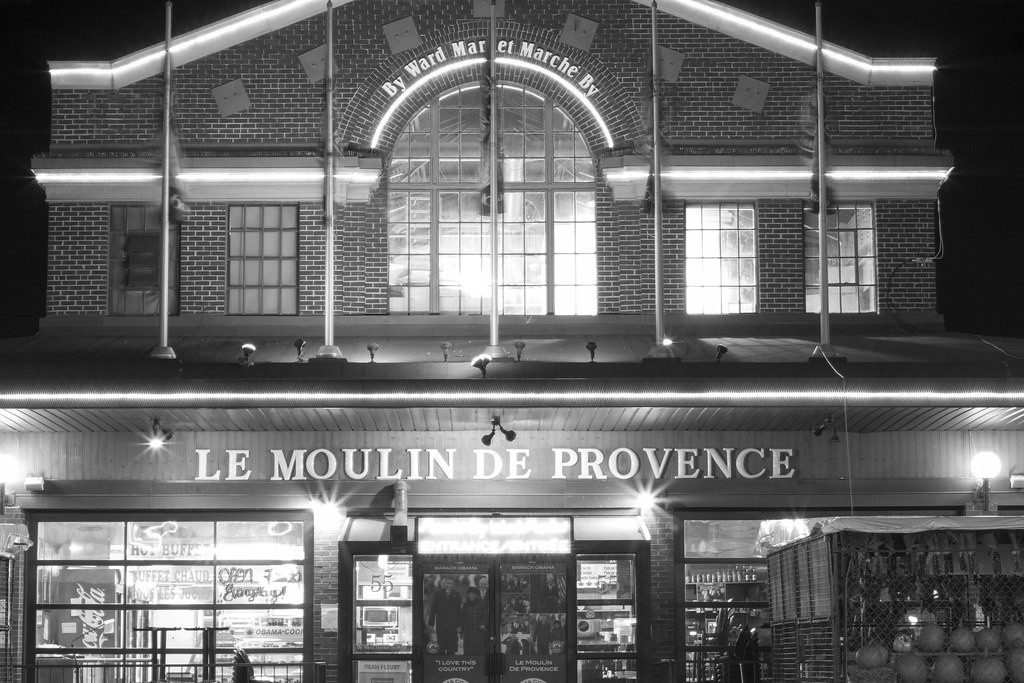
510,617,527,634
531,614,566,654
542,572,560,599
501,574,528,593
423,574,490,655
504,635,530,655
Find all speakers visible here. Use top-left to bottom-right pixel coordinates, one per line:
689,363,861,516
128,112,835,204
25,478,44,490
